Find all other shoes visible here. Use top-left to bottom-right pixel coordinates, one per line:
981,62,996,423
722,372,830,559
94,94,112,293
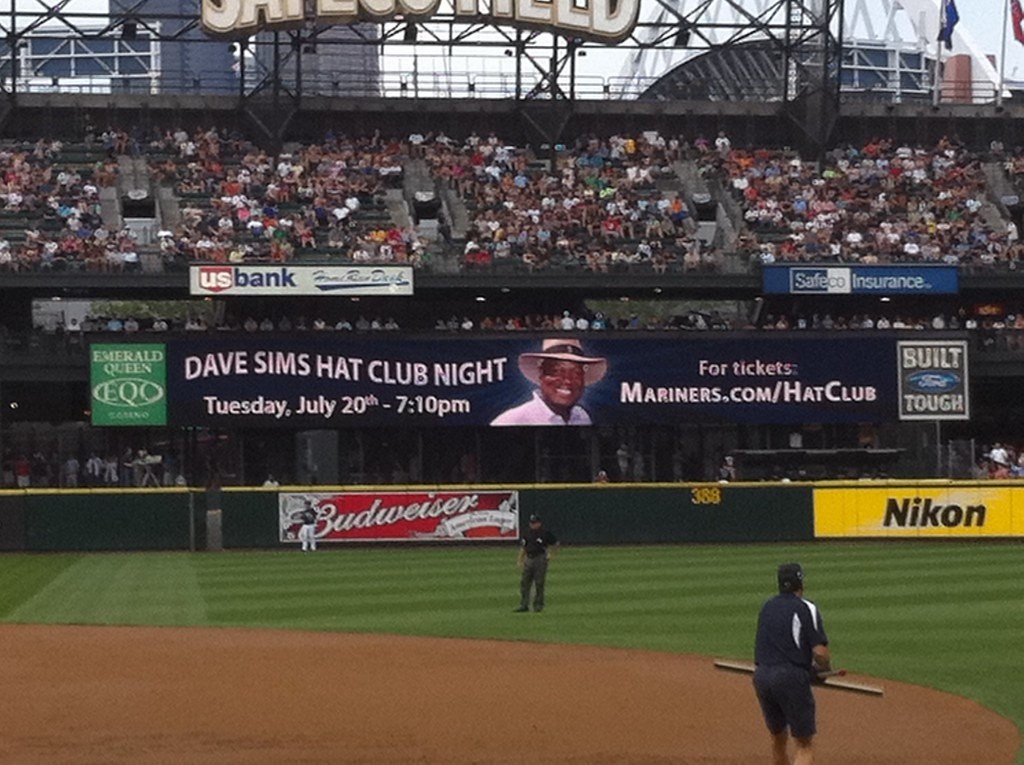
516,606,528,612
534,604,542,612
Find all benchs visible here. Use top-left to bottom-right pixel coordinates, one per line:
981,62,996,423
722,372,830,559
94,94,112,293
0,144,402,255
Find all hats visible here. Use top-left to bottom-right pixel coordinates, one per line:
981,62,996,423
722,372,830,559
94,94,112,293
528,514,541,521
516,338,608,387
778,563,803,585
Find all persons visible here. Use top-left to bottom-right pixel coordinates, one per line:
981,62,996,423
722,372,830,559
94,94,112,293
0,307,1024,488
1,112,1024,274
489,339,607,427
512,510,559,612
753,562,830,765
291,501,319,552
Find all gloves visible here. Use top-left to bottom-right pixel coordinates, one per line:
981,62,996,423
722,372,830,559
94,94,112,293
809,665,826,686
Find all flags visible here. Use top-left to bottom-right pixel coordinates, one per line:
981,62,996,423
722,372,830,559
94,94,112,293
1011,0,1024,46
938,0,958,51
